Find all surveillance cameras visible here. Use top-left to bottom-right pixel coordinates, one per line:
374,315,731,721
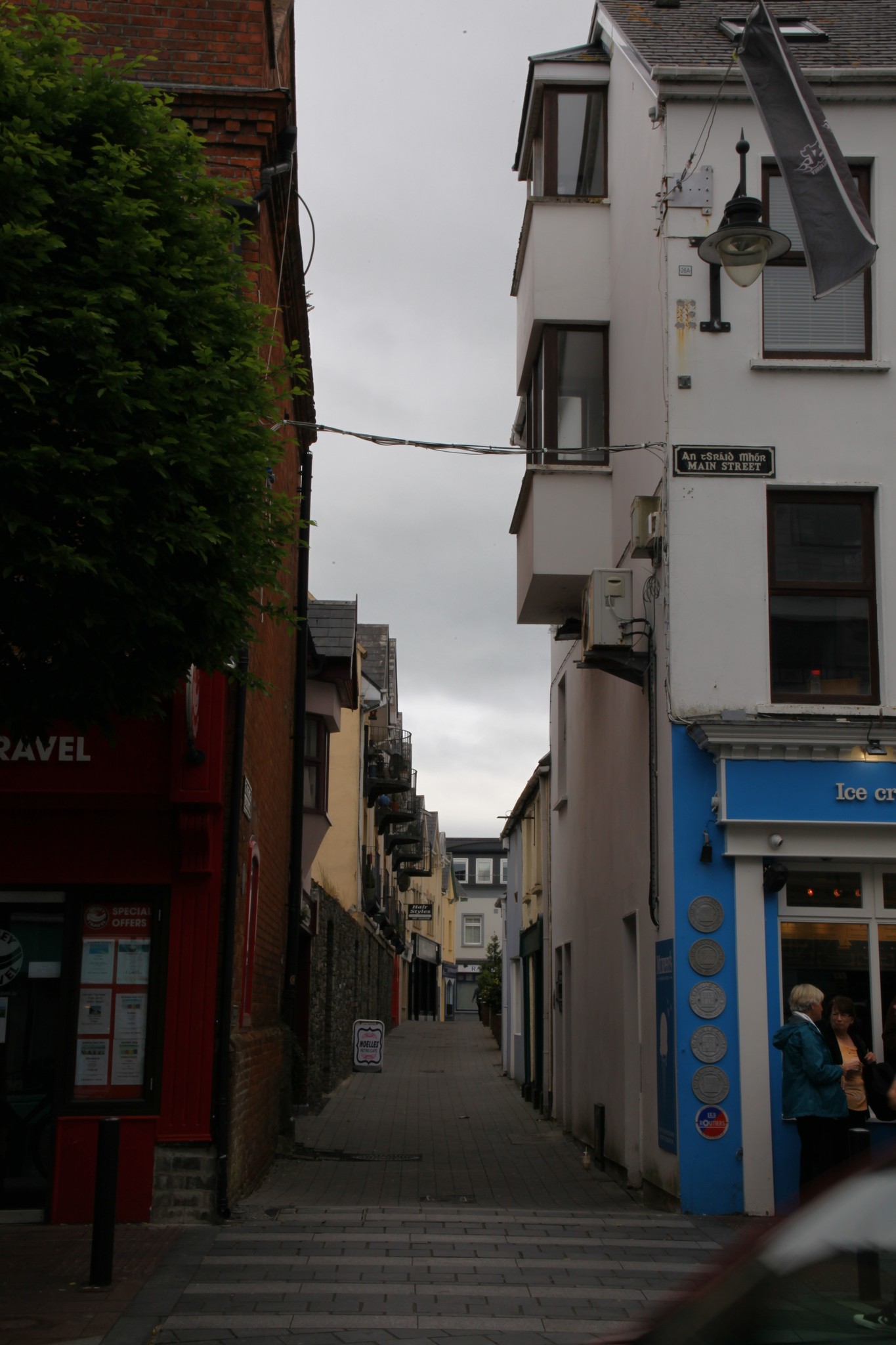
768,835,783,849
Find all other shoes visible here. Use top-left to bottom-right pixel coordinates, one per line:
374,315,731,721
854,1304,896,1332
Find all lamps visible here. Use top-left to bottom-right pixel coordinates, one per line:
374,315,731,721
689,127,792,332
554,619,582,641
866,739,888,755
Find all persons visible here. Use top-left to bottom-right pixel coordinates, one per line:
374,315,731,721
838,1034,851,1053
772,982,848,1205
826,993,877,1172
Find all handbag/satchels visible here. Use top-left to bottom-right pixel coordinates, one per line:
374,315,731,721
862,1061,896,1121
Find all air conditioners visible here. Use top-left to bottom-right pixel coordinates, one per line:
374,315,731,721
582,567,633,660
